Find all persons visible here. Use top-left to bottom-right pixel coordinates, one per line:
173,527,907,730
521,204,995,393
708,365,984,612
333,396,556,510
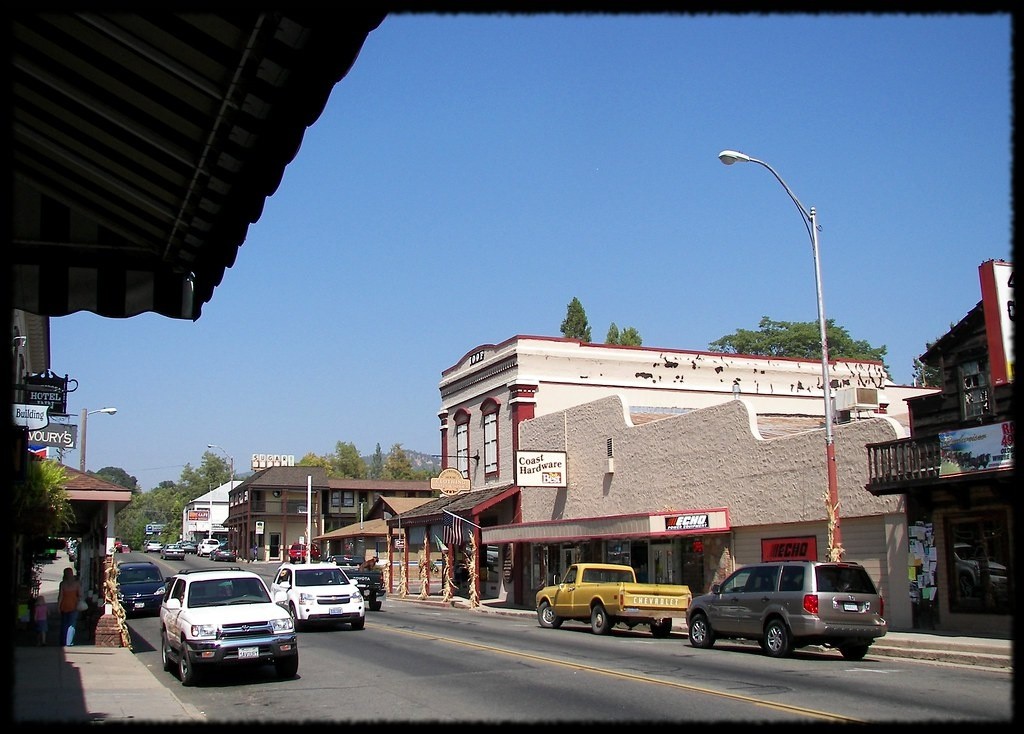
55,567,85,646
34,596,52,644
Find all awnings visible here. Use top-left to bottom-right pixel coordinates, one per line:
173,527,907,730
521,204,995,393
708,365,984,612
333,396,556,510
0,0,383,324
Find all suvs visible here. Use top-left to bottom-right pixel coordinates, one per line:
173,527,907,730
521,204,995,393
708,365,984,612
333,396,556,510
683,559,888,661
115,536,122,553
288,542,321,564
161,567,300,687
271,562,366,630
197,539,220,557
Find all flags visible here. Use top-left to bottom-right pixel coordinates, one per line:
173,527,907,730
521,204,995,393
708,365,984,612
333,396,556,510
441,512,462,546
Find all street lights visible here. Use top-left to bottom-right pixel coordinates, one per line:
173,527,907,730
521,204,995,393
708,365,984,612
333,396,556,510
80,407,117,472
207,444,233,490
718,149,843,563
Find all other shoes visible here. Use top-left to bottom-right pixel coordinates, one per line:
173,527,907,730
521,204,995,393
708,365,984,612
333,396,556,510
32,641,40,645
66,643,74,646
42,642,48,646
57,638,64,645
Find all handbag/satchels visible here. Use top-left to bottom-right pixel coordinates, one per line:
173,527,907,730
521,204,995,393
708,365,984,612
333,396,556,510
76,596,88,611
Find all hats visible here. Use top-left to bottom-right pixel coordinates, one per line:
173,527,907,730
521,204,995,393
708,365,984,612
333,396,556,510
35,596,48,605
64,568,73,576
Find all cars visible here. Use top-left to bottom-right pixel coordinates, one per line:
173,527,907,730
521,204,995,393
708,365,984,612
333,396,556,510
487,546,498,565
220,541,228,549
143,540,163,553
210,547,236,562
953,543,1008,594
179,541,197,554
160,543,185,561
66,537,75,562
115,560,166,617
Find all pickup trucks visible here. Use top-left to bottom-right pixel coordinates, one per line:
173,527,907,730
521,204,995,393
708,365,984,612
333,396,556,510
536,563,692,637
325,555,388,612
